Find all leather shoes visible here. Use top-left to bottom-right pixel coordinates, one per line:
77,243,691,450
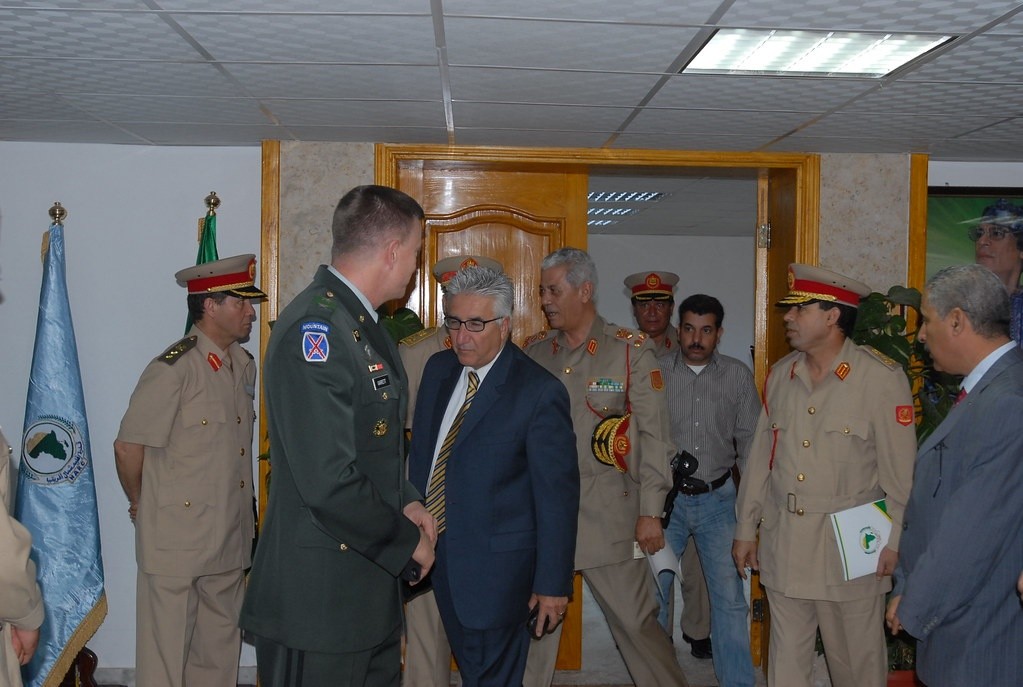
683,633,715,658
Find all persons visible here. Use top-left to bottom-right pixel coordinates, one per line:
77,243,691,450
886,266,1023,687
112,252,268,687
409,264,580,687
621,270,713,658
0,434,44,687
730,264,918,687
236,185,499,687
966,205,1023,346
397,256,503,687
654,295,763,687
518,245,689,687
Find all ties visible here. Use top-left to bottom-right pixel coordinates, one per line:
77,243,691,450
947,386,967,416
426,371,481,534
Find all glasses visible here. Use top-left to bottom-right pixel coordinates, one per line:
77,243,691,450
444,316,502,333
968,224,1023,241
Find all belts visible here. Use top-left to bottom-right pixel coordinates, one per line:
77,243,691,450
673,468,731,494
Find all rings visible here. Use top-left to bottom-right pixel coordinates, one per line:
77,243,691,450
558,613,564,616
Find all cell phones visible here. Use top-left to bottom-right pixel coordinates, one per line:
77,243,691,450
527,608,550,641
402,557,422,582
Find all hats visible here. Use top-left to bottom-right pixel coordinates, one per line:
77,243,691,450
774,263,872,309
433,256,504,291
979,197,1023,229
175,255,268,299
590,412,641,483
623,271,679,301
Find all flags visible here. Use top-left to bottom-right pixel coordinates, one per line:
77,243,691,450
194,211,260,591
11,222,108,687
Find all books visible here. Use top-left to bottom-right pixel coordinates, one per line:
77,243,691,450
831,499,894,581
